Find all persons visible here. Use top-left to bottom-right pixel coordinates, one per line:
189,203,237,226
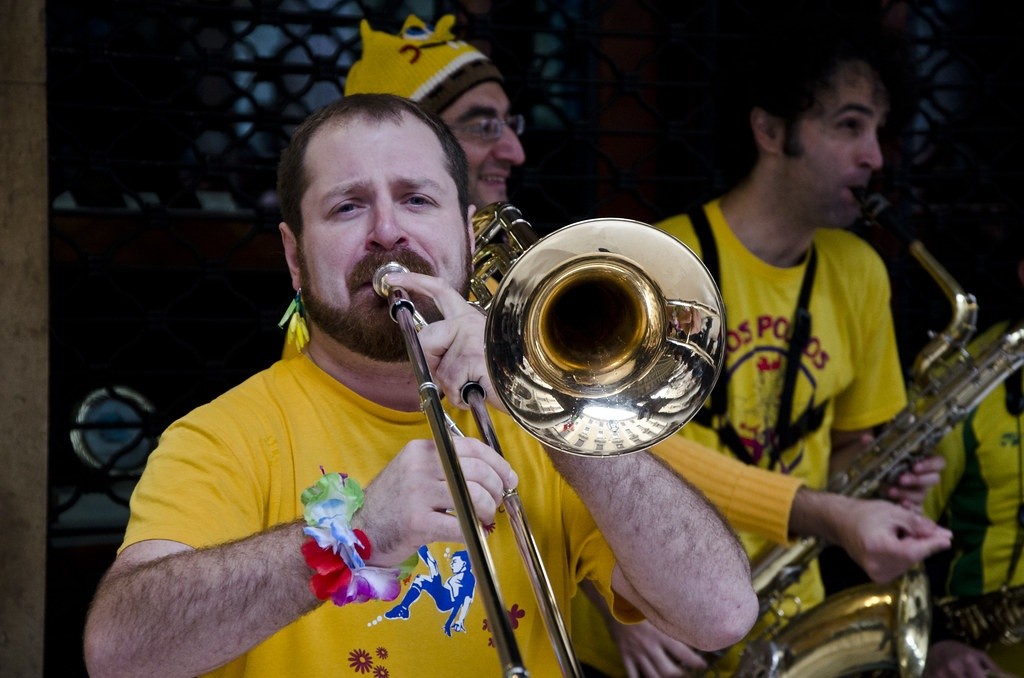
652,26,952,678
282,15,708,678
922,253,1024,678
85,93,759,678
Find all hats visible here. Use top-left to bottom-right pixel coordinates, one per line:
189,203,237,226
344,16,504,117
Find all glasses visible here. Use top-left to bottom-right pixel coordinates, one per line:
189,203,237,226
448,113,524,142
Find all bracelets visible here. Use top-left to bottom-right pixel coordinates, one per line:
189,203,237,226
301,473,419,607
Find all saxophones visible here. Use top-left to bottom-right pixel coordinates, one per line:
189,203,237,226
669,187,1023,678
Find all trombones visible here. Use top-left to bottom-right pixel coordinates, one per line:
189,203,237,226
371,201,728,678
